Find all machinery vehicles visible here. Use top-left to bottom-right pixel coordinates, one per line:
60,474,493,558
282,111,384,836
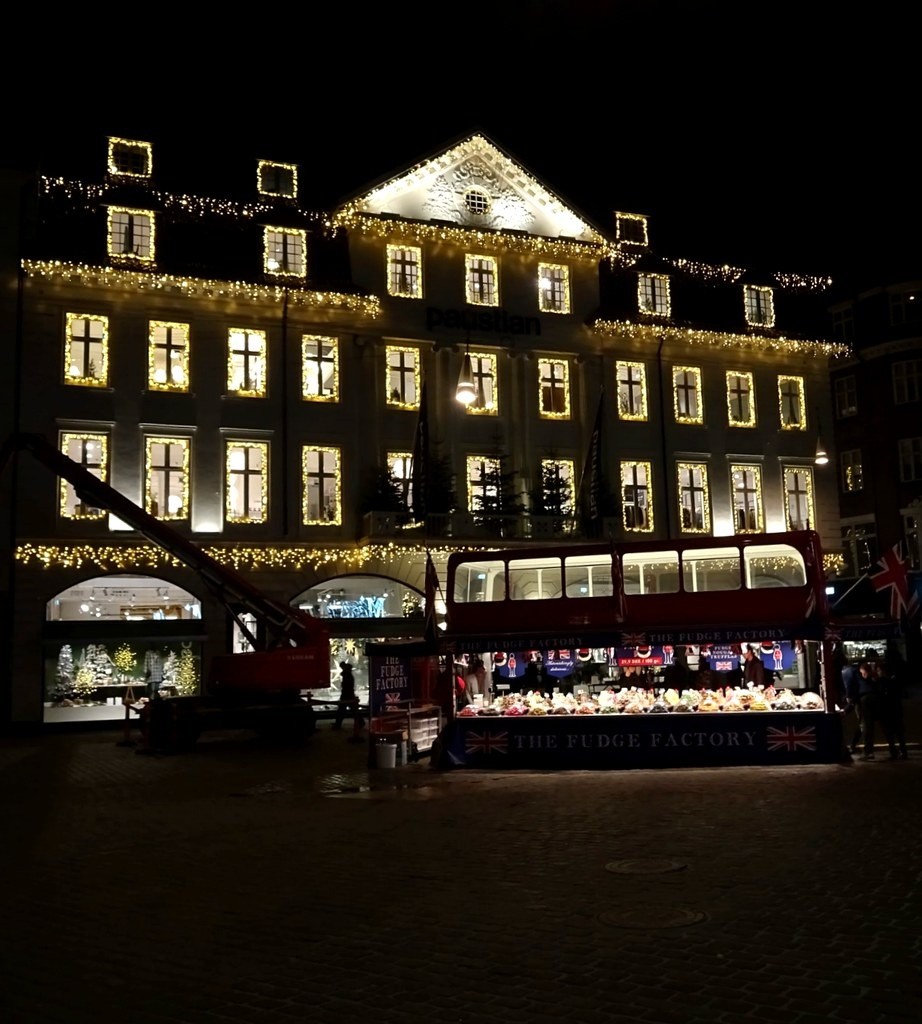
29,430,330,759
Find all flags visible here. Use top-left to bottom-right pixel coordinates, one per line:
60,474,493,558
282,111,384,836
420,560,440,654
866,537,920,625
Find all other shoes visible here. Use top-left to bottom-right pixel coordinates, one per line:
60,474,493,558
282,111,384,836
860,754,875,762
888,755,908,760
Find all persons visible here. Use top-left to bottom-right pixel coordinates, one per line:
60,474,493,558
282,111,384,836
841,646,908,759
331,663,366,731
505,654,713,691
463,657,488,703
141,646,163,700
739,645,764,687
